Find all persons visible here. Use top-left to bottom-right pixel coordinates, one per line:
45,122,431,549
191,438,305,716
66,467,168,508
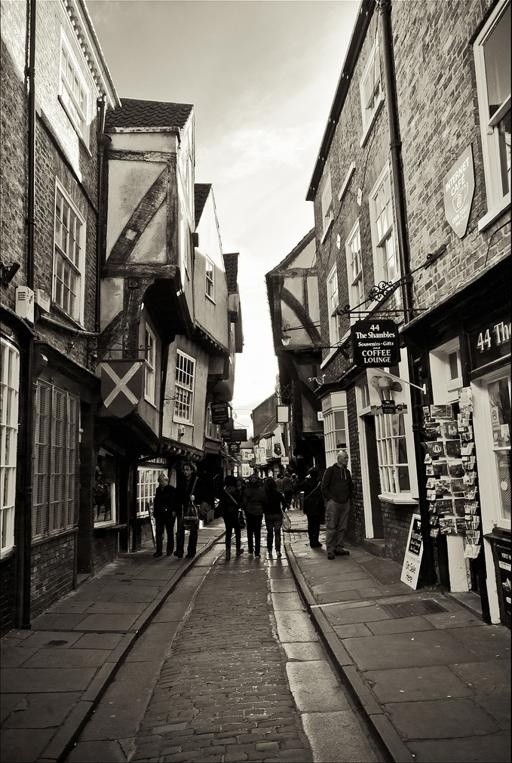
217,472,299,560
303,466,326,548
321,448,354,559
152,472,177,558
172,462,202,559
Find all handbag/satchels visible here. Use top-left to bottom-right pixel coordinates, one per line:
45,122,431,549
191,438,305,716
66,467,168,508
238,509,246,528
181,504,200,529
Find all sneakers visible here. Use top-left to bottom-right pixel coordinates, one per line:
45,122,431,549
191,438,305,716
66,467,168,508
226,549,282,561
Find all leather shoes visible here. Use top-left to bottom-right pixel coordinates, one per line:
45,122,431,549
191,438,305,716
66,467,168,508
153,551,195,558
327,546,349,560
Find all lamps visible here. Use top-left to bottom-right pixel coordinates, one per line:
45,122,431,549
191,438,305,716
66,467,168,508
281,320,321,347
